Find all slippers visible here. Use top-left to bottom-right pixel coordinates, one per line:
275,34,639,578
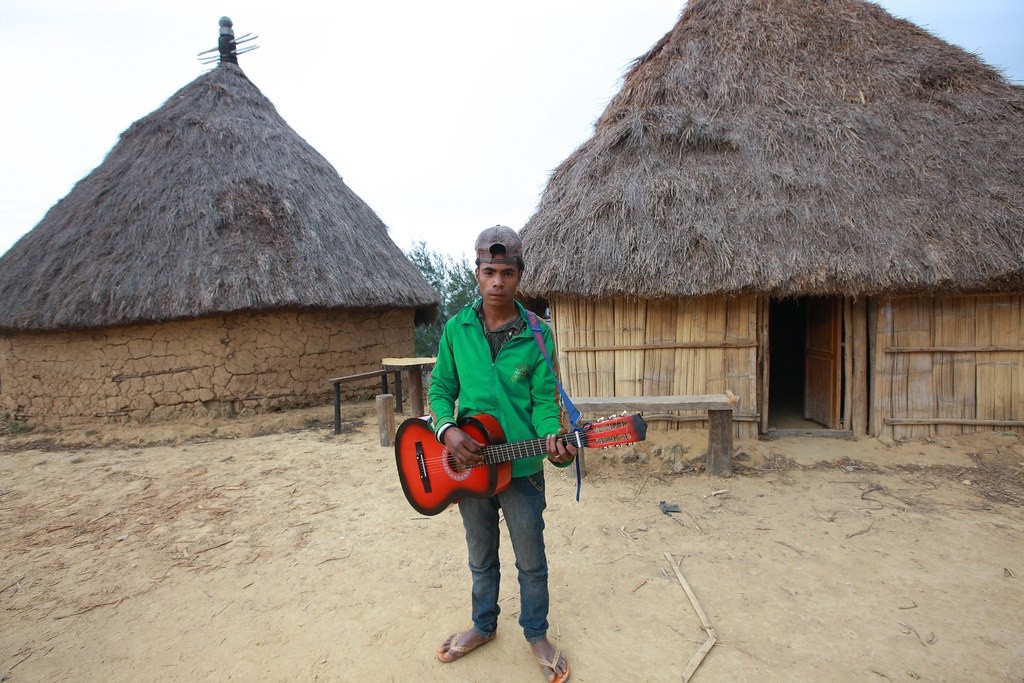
531,644,569,683
437,629,497,662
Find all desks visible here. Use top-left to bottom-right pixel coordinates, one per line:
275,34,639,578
382,357,437,419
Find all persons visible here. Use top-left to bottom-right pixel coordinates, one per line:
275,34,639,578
421,222,580,682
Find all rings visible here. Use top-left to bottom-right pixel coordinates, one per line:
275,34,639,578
457,455,463,458
554,454,561,458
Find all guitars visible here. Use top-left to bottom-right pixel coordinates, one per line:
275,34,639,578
394,410,648,517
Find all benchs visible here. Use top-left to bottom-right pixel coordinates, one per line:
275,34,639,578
562,394,741,479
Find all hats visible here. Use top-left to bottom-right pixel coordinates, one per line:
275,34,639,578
475,225,522,264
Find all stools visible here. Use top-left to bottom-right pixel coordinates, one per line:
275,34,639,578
376,394,396,446
